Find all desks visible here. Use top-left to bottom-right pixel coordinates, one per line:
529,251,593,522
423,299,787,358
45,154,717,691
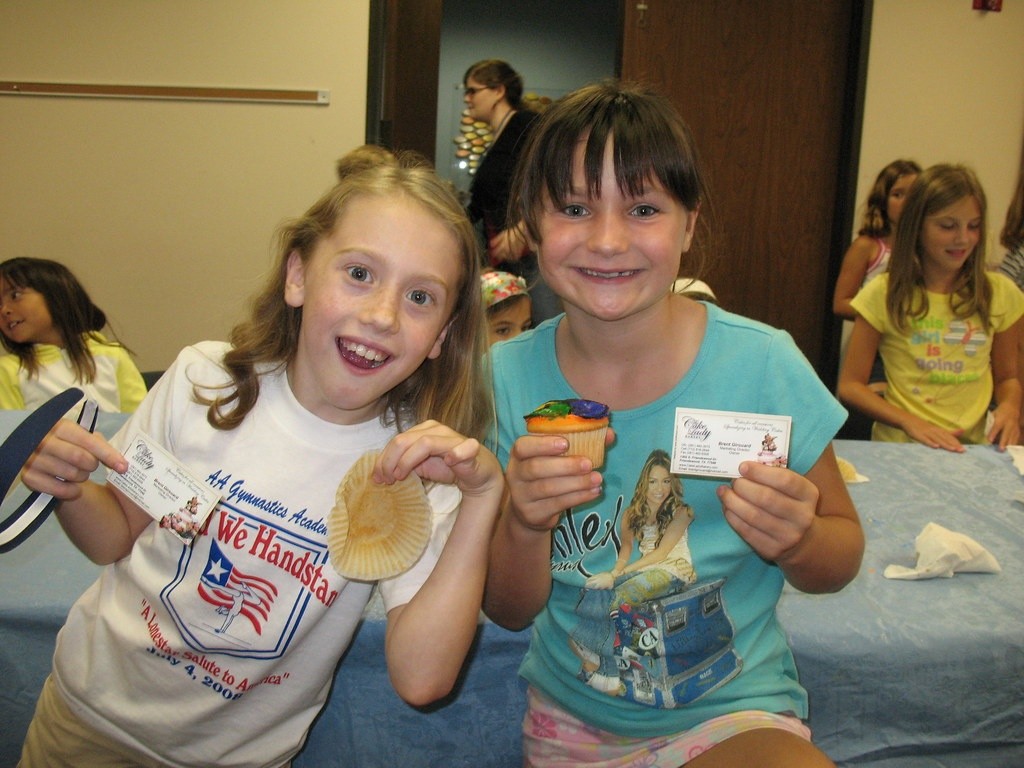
0,407,1024,767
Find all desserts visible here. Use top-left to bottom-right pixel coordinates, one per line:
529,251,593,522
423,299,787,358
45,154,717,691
522,399,609,470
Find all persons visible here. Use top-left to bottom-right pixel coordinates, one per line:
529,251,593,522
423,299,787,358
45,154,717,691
462,59,867,768
832,159,1024,453
0,256,147,413
18,145,505,768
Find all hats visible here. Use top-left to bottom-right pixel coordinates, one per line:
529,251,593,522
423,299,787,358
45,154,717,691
669,278,718,305
480,271,531,313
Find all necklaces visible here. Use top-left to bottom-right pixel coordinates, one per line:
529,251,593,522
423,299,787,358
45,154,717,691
490,108,514,143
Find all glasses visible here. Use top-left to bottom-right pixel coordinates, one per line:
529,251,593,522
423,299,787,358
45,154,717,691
465,86,490,96
